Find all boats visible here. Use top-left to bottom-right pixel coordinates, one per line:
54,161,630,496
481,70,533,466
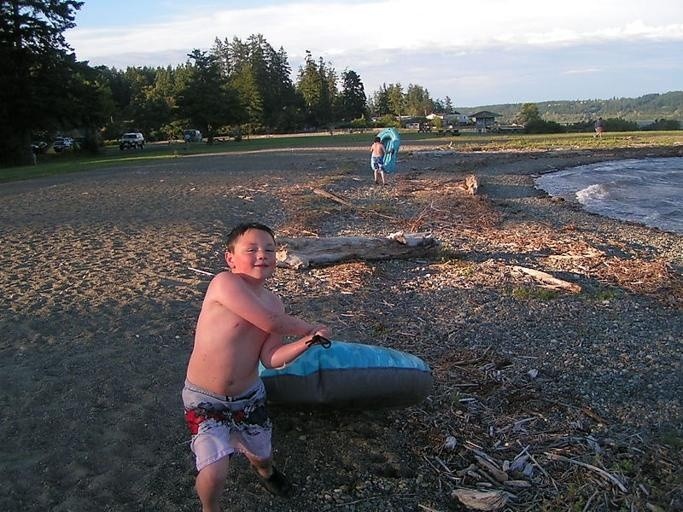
258,338,436,411
370,128,400,174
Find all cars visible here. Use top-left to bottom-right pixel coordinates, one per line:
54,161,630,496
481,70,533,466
31,141,48,152
54,138,72,153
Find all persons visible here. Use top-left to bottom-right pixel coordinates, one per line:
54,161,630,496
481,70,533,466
31,146,37,165
493,121,501,133
449,123,454,136
416,119,436,134
594,117,603,138
181,224,332,511
370,137,388,186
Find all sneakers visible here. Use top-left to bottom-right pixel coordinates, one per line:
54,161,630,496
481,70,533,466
250,464,292,495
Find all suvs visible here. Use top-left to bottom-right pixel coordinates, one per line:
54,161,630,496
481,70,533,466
120,133,146,151
184,129,203,143
